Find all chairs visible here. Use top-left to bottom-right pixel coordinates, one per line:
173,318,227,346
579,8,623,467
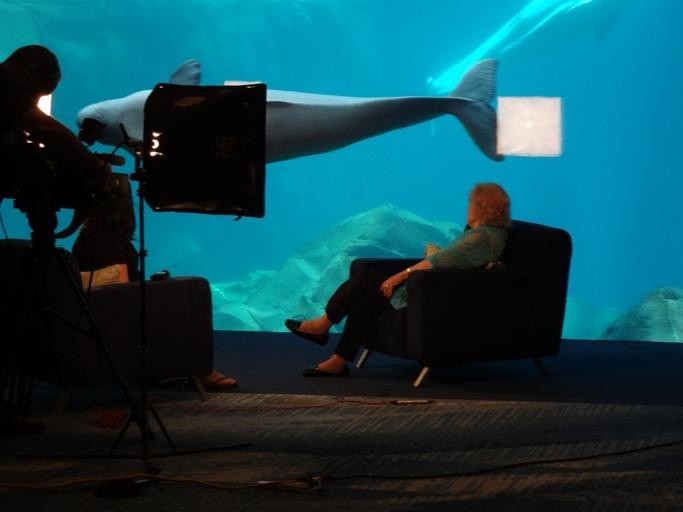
350,219,573,387
0,238,214,416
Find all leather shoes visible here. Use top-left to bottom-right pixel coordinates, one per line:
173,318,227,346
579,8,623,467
284,318,331,346
300,364,352,378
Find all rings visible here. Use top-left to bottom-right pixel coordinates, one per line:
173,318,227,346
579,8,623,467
384,284,389,288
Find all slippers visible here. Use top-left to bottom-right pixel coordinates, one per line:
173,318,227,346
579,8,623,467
207,374,240,392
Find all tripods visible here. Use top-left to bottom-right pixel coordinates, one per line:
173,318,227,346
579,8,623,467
108,145,169,471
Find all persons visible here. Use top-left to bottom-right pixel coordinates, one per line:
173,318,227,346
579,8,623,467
283,180,512,382
0,45,63,214
73,172,240,394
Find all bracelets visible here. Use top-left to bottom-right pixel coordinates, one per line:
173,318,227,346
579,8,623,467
406,267,411,277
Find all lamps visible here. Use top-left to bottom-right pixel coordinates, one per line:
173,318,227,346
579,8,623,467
137,83,267,280
